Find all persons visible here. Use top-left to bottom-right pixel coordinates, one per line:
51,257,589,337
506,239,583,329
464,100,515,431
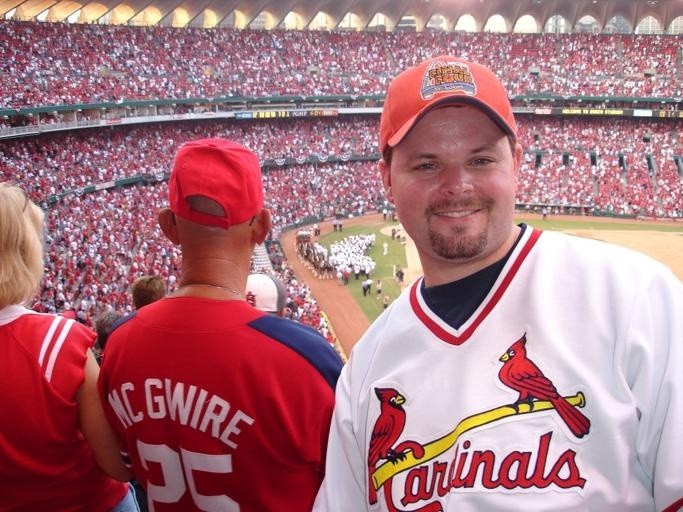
305,52,682,511
0,183,141,512
95,135,345,511
0,13,681,373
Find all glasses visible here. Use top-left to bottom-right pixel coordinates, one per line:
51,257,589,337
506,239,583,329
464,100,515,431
0,172,29,213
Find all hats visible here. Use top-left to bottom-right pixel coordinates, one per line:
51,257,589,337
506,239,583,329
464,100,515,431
380,54,516,152
169,139,263,226
246,273,287,313
